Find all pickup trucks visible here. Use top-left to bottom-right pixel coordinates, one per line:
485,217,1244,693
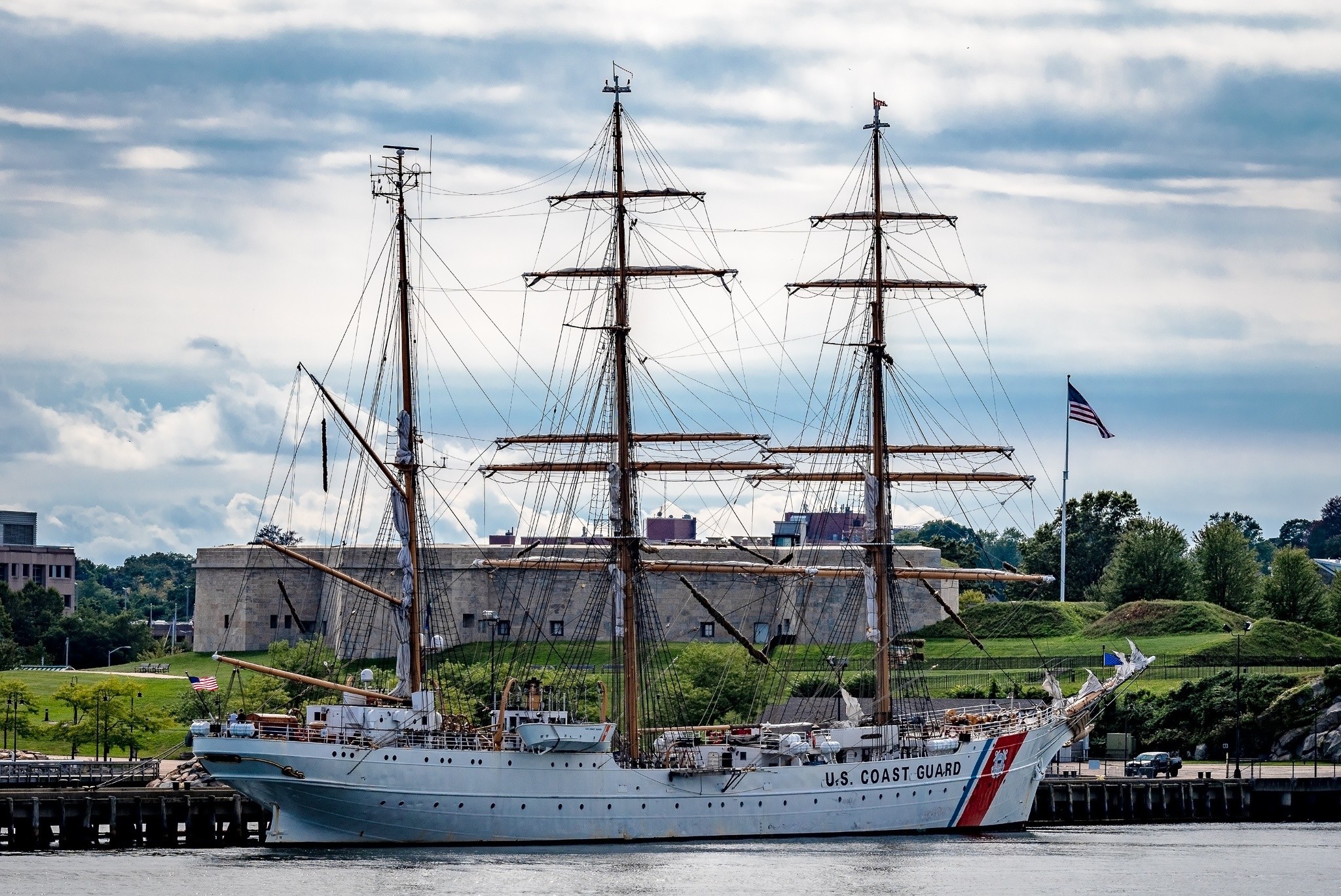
1125,752,1182,779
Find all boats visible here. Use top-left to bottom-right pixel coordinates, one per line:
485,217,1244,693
514,721,618,756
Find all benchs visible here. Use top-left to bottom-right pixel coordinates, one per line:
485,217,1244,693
134,662,171,673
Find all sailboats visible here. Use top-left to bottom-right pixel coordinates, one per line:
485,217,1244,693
183,57,1159,853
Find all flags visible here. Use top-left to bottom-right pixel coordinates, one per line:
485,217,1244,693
1068,381,1116,439
188,676,218,690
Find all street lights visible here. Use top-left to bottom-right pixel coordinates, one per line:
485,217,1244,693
108,645,132,667
130,687,143,763
1123,697,1135,776
70,675,80,753
652,688,663,753
74,582,78,616
96,689,109,761
185,585,191,622
212,692,221,737
122,587,130,612
987,692,1000,712
1222,622,1254,778
481,609,501,710
826,656,849,720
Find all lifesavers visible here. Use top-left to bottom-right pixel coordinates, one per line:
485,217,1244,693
710,731,723,744
947,709,956,716
185,730,192,748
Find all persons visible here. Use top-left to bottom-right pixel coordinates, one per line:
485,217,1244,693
230,708,247,725
